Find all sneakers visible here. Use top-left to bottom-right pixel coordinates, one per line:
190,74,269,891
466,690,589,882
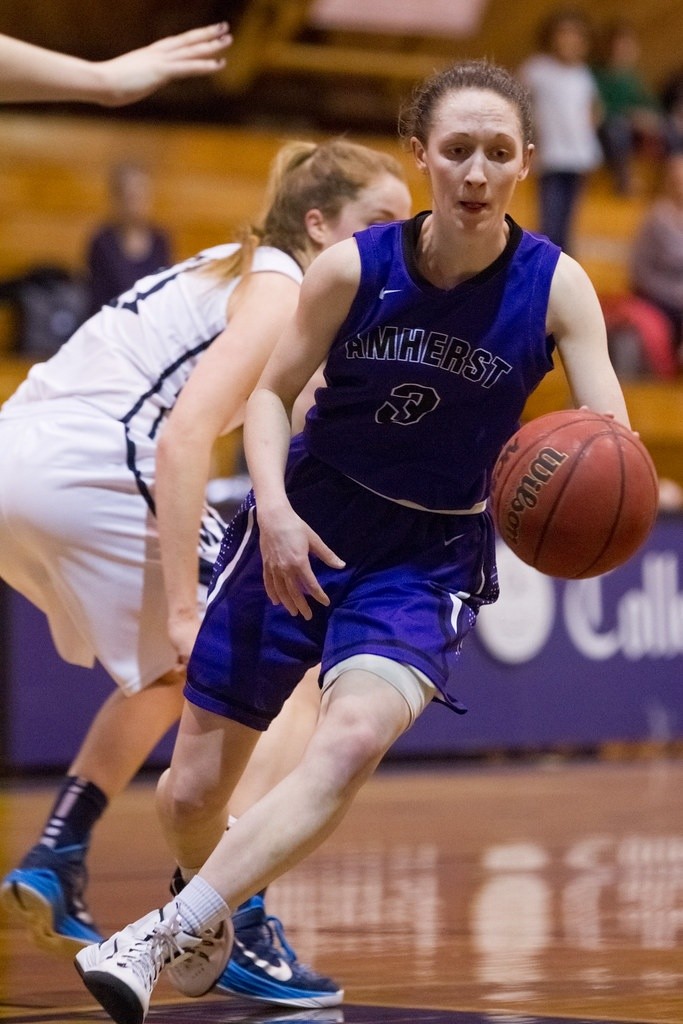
0,843,104,961
169,869,345,1009
169,813,237,997
73,900,202,1024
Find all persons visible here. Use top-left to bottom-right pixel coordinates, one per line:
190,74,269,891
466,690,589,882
74,12,683,1024
1,138,440,1024
0,23,232,107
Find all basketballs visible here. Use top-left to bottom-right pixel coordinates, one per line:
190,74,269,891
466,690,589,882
488,406,661,582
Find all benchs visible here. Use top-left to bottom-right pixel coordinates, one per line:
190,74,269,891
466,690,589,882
0,91,683,486
